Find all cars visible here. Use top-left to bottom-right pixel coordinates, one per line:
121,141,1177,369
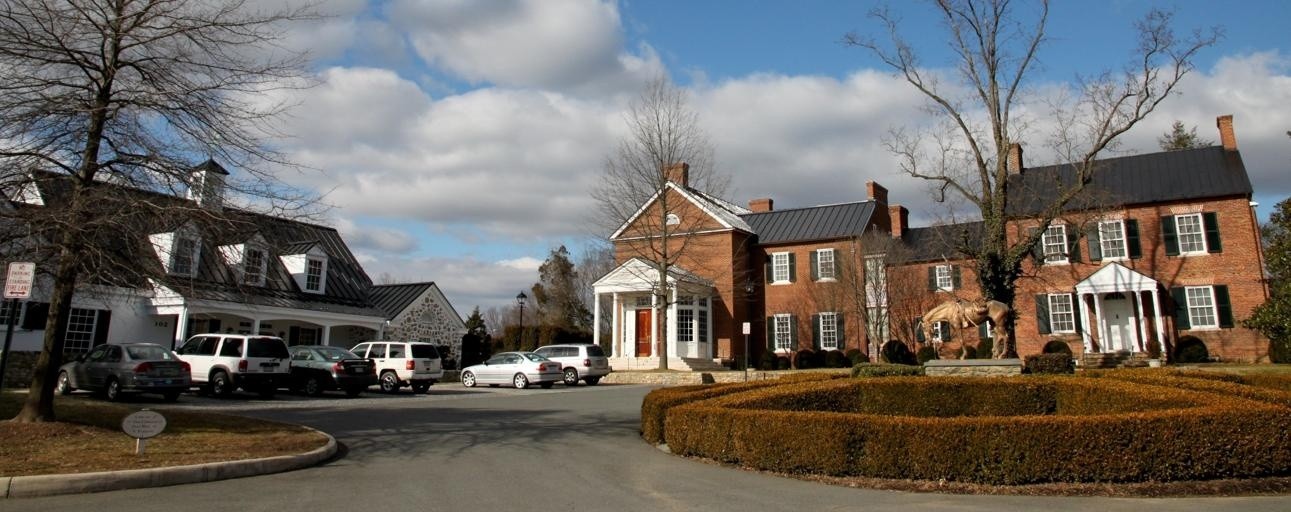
57,342,193,404
287,344,379,398
459,351,566,390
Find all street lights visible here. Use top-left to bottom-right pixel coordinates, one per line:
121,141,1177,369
516,291,528,350
741,276,760,382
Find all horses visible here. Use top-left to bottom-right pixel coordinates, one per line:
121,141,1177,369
914,296,1021,360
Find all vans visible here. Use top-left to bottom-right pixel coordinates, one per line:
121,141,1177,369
349,340,444,395
170,333,292,399
532,342,609,387
